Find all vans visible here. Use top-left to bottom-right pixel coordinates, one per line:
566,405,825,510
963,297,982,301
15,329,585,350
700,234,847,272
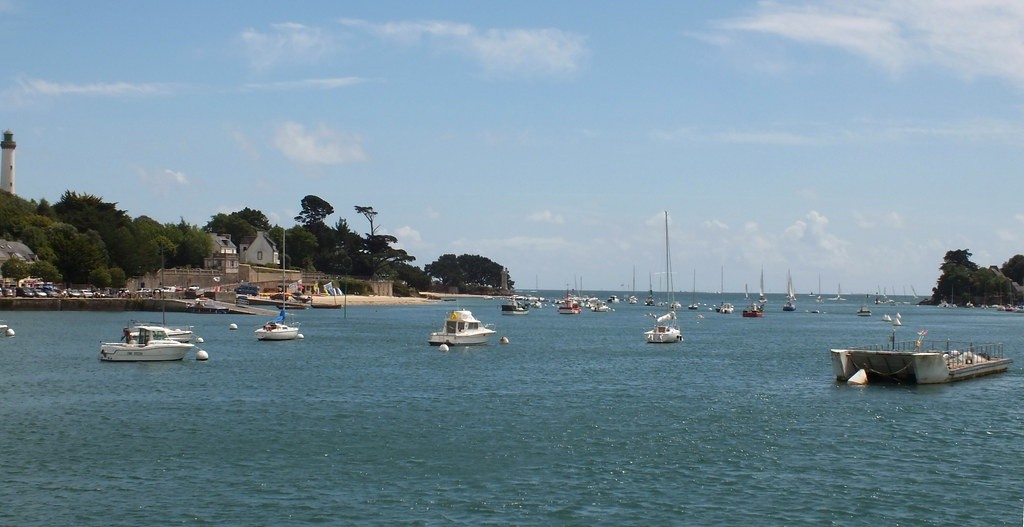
234,284,261,297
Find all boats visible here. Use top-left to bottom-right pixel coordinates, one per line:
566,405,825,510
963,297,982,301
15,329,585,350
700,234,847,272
431,310,498,347
310,299,342,309
828,329,1014,387
277,304,305,309
99,328,195,362
485,290,551,316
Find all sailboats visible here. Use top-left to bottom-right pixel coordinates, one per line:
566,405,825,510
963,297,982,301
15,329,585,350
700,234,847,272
855,284,1024,326
253,232,302,340
687,266,846,319
123,243,193,343
553,209,683,343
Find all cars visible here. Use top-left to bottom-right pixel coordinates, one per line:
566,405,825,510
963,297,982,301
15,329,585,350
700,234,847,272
270,293,288,301
0,284,200,302
